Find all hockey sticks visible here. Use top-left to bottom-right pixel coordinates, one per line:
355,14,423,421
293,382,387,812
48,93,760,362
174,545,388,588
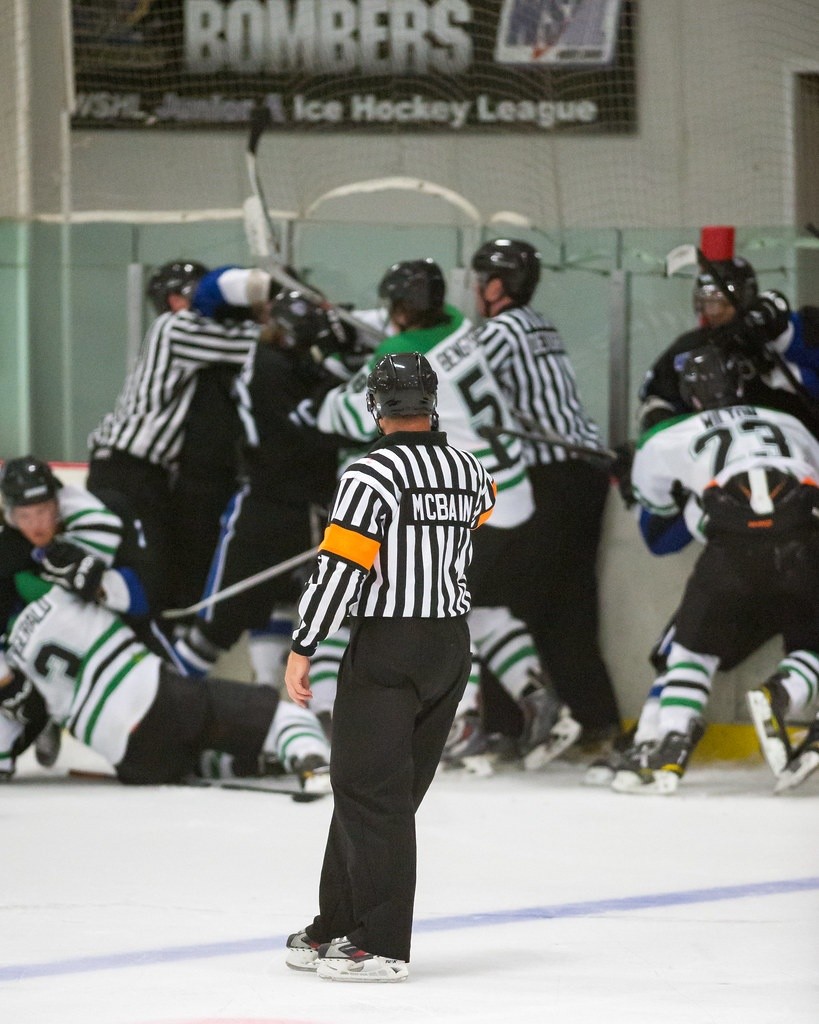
665,244,819,414
477,423,617,459
161,546,319,618
69,769,324,803
241,103,284,267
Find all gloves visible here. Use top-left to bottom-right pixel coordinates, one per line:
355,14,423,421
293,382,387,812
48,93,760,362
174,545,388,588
37,544,108,598
611,440,640,511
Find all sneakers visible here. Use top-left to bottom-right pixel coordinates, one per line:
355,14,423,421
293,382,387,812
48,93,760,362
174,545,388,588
314,934,408,984
608,714,708,794
285,925,321,974
584,730,634,785
292,755,333,798
770,721,819,796
738,685,795,778
437,712,505,776
513,687,582,772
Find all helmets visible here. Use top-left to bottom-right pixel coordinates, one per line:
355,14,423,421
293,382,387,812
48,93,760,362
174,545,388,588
366,350,439,420
0,455,65,506
148,259,209,312
271,284,333,352
691,257,759,315
679,344,746,413
464,238,542,304
378,258,446,321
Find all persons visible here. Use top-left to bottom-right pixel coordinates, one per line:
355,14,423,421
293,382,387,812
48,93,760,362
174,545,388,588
284,351,498,965
0,236,819,787
